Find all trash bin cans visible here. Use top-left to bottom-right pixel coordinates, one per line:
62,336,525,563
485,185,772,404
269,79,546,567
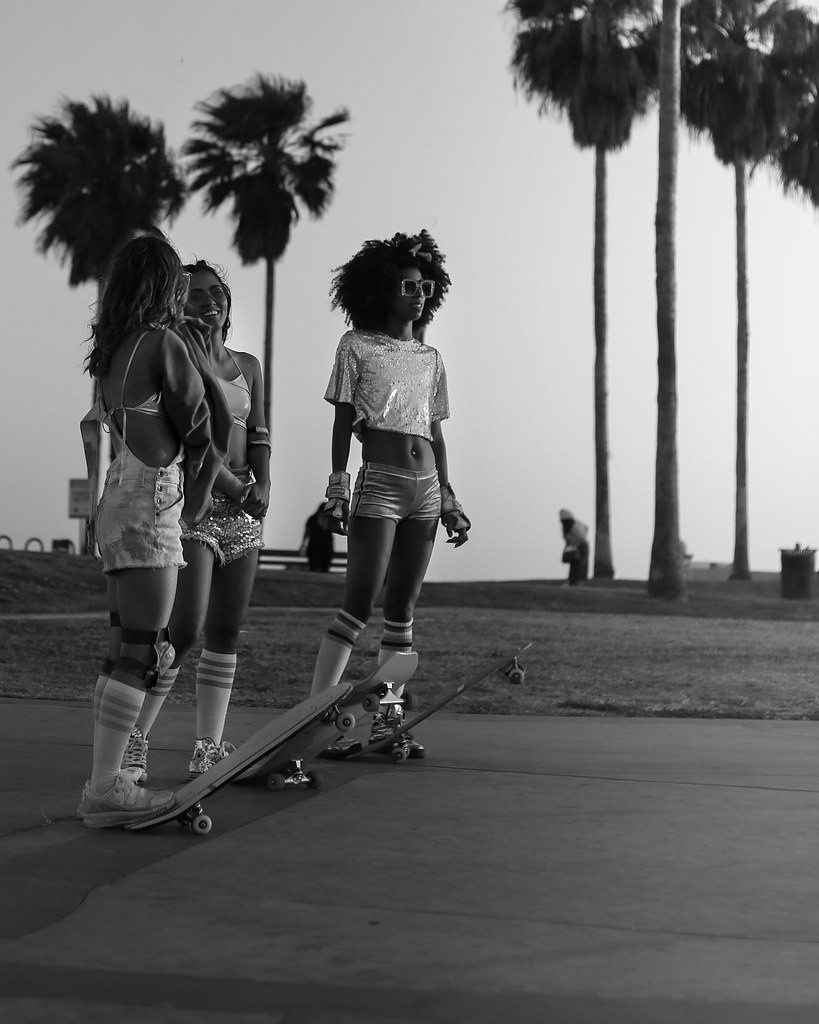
779,549,816,600
52,539,75,554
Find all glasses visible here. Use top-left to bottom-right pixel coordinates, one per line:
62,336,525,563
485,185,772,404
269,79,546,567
397,279,435,298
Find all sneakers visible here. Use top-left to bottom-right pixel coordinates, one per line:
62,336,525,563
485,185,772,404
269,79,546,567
321,736,363,757
123,725,150,782
78,769,177,828
368,704,425,759
187,737,235,781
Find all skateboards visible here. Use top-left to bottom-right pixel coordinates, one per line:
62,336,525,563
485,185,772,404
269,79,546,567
232,647,419,791
123,681,355,836
343,640,534,763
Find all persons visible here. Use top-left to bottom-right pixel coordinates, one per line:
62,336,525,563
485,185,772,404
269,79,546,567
310,227,471,756
78,236,232,826
560,510,589,580
121,260,272,784
301,502,346,572
